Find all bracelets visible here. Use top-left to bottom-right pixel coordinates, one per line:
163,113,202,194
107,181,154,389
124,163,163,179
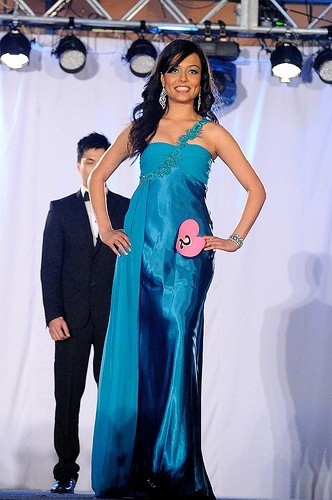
229,234,244,249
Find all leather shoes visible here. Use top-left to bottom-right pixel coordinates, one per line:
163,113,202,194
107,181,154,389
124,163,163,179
50,478,75,494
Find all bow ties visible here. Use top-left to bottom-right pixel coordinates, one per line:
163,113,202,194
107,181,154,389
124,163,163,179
83,191,90,201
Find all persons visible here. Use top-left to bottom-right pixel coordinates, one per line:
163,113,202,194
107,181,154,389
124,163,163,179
87,40,267,500
40,130,130,494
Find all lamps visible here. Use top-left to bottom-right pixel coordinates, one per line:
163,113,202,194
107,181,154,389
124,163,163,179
56,36,86,73
126,39,157,78
313,48,332,85
0,29,31,70
271,42,303,83
